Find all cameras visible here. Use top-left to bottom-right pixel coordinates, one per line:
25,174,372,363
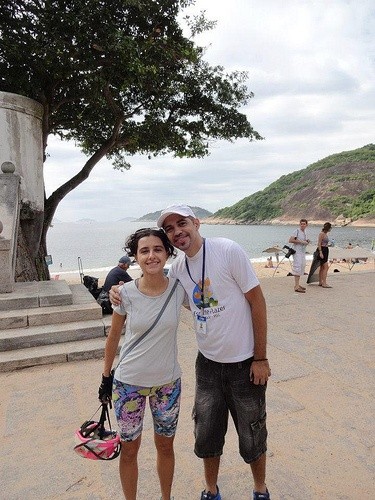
282,245,296,258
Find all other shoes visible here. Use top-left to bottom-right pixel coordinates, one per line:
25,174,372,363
295,288,305,293
299,286,306,290
253,487,270,500
201,483,222,500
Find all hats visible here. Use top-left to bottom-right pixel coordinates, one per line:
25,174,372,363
157,204,196,229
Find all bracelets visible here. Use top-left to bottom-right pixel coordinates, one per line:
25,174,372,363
253,359,269,362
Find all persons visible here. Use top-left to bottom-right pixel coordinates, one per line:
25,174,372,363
102,255,134,290
306,222,333,288
97,226,190,500
288,219,311,293
109,203,272,500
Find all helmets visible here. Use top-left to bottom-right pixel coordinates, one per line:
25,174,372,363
73,419,121,461
